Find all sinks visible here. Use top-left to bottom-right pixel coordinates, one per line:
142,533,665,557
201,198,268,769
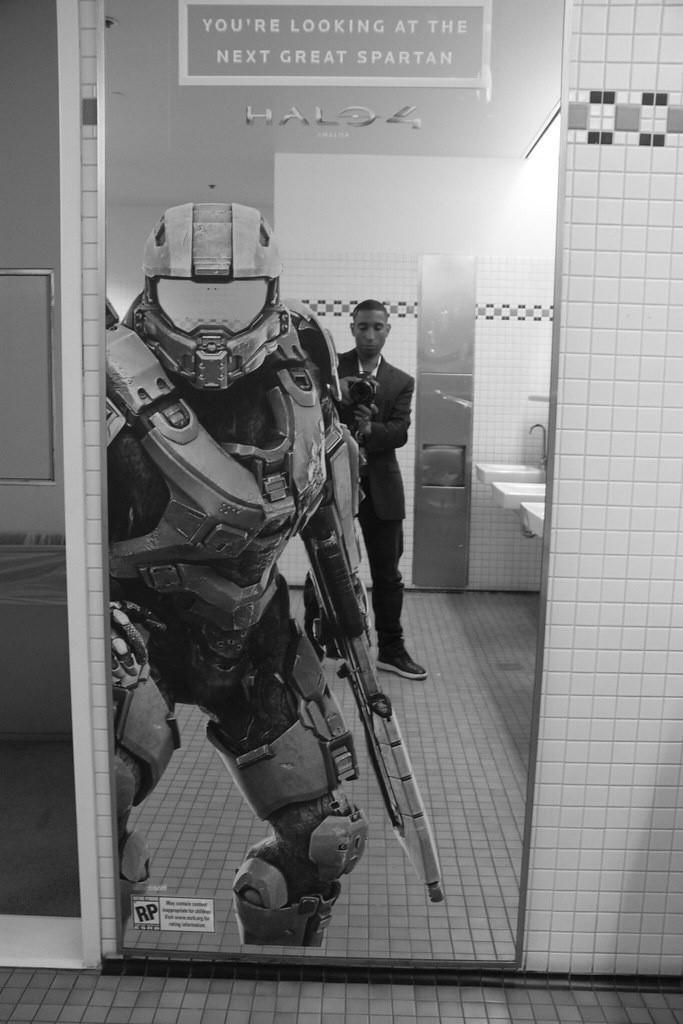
490,479,546,510
474,461,546,486
519,499,547,542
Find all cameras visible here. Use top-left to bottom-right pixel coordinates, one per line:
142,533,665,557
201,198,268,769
342,374,381,412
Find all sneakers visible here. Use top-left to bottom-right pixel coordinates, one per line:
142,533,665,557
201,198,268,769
375,639,428,679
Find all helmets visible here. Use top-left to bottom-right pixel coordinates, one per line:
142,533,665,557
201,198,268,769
142,202,290,393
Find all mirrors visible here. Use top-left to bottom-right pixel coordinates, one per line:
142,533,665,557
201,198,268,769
80,1,575,976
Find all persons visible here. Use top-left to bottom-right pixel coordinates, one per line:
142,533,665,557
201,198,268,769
301,298,430,682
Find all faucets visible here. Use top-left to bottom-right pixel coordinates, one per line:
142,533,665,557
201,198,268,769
528,423,547,470
